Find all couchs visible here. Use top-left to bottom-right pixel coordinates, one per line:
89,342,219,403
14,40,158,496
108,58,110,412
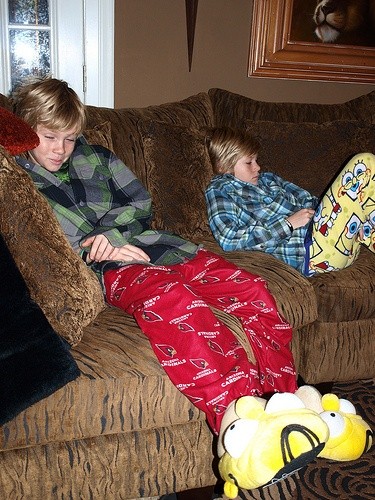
0,88,375,500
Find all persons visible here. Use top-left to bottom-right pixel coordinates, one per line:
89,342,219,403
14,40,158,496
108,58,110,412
13,78,298,434
203,127,375,277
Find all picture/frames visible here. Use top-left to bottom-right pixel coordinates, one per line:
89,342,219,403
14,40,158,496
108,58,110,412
247,0,375,84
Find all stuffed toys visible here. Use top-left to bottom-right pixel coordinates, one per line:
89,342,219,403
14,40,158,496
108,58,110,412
216,383,373,496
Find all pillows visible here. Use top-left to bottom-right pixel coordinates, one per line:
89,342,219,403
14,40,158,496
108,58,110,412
0,143,110,351
0,107,40,157
242,119,370,204
138,117,218,243
82,121,116,156
0,234,80,428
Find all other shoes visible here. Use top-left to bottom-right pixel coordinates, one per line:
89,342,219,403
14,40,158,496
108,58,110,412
218,386,372,499
